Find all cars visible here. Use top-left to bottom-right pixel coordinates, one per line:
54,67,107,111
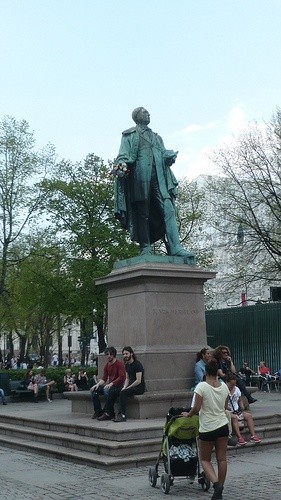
24,354,39,364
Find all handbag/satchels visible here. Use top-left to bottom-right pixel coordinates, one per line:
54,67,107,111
234,409,245,420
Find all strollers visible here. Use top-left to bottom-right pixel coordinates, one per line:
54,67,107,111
149,407,211,494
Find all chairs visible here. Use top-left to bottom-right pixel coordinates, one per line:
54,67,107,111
237,374,281,392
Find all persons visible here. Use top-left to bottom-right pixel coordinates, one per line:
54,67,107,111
97,346,145,422
182,346,281,500
0,348,97,406
90,346,125,420
113,107,196,257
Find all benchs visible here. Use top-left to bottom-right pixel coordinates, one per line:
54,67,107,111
9,378,54,402
63,377,97,401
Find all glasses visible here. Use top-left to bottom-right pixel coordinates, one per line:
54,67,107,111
222,351,227,354
105,352,112,355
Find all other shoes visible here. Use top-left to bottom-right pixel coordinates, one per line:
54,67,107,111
238,437,247,445
248,395,258,404
250,435,262,443
211,480,224,500
96,411,115,420
92,410,102,419
112,413,127,422
